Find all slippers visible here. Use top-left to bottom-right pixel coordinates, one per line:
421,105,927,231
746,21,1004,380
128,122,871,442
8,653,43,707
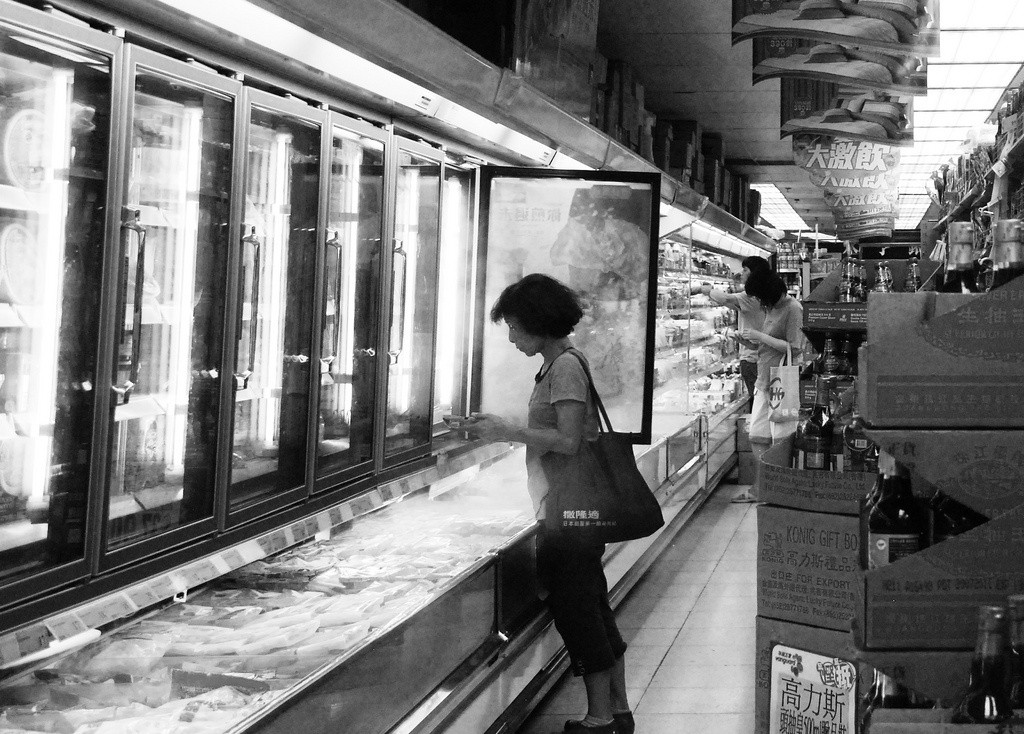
731,488,758,503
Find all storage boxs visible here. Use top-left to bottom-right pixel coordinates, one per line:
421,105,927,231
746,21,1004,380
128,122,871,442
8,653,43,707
860,430,1024,650
754,504,866,636
753,616,858,734
755,390,882,514
854,277,1024,427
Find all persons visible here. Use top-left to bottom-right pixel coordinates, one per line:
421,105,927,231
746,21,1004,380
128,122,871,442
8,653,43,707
449,272,638,734
728,268,807,504
699,256,774,403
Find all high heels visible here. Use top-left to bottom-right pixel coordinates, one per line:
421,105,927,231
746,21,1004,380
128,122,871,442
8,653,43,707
611,711,635,734
563,718,618,734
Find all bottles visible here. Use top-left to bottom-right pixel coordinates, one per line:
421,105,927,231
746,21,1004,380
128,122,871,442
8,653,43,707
855,591,1023,724
837,221,1024,313
863,452,976,568
794,329,878,473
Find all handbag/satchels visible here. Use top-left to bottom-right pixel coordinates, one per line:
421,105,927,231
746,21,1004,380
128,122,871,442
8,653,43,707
538,350,665,545
766,340,801,423
